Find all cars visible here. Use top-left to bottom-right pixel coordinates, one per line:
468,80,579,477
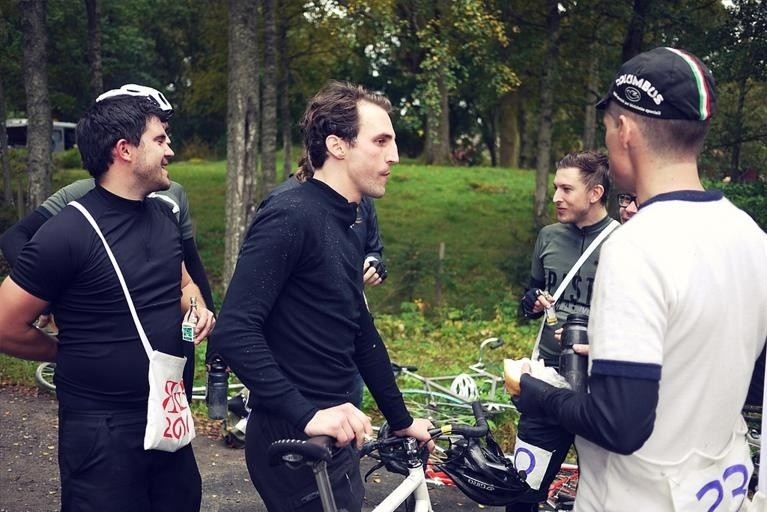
6,118,77,151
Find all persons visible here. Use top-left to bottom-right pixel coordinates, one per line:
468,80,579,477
522,155,621,364
0,96,217,511
1,82,212,404
505,47,767,511
618,189,639,224
212,82,439,512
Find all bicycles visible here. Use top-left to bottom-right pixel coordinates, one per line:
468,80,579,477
36,362,251,449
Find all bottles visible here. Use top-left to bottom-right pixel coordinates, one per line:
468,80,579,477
559,312,590,404
205,358,229,419
542,292,557,327
181,297,202,343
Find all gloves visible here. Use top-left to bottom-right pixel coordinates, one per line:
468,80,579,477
511,372,558,417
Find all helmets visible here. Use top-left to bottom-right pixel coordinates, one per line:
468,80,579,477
436,438,539,506
376,420,429,476
93,84,176,122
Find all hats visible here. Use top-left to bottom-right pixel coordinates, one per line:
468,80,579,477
593,46,717,121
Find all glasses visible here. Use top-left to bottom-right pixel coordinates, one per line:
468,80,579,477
616,193,640,210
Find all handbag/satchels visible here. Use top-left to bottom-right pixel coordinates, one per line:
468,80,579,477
143,350,196,453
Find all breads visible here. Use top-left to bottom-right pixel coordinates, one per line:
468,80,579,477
501,355,560,396
